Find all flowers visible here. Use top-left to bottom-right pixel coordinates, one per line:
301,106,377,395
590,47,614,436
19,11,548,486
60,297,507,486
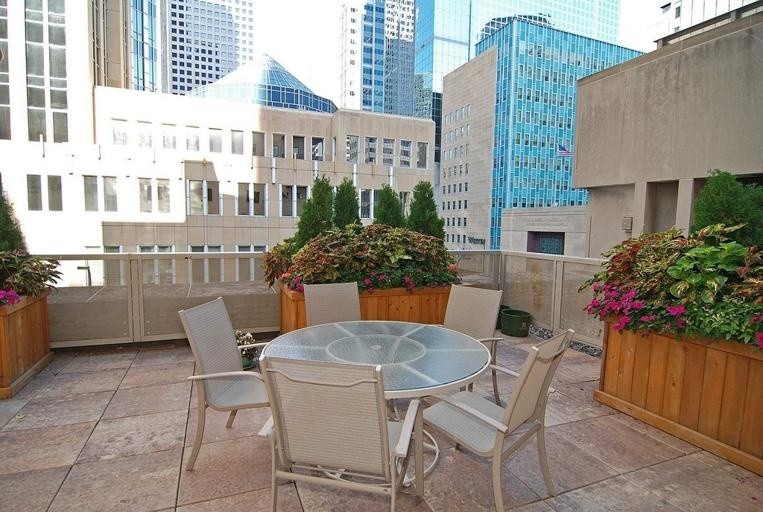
575,223,763,355
282,224,463,294
2,248,63,307
233,329,262,361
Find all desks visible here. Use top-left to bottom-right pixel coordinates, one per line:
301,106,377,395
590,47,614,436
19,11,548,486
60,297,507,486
260,320,492,490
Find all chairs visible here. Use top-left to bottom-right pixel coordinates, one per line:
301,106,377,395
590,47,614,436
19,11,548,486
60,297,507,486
259,353,420,510
304,283,362,327
422,327,574,512
178,296,271,473
444,282,503,405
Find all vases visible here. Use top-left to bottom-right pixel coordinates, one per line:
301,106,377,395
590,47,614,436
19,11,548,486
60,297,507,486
494,304,511,329
280,284,450,335
242,349,260,370
